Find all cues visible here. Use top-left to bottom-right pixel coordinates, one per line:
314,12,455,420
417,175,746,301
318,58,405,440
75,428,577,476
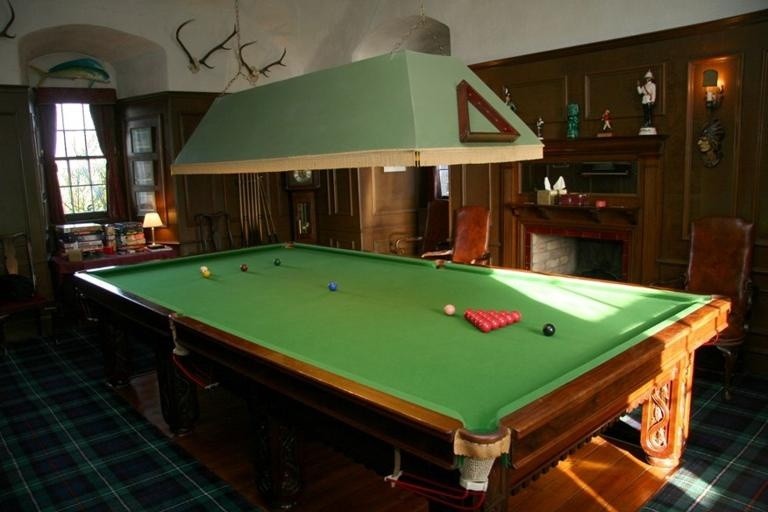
238,173,278,246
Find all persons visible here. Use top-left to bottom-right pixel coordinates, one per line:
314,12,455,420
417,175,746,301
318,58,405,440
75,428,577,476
636,69,657,127
601,110,612,131
536,117,543,137
503,86,518,112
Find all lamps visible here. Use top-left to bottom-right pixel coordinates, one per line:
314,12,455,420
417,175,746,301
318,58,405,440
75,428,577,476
142,212,166,249
703,70,718,101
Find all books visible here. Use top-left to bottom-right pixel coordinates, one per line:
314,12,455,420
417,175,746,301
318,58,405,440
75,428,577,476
55,220,147,258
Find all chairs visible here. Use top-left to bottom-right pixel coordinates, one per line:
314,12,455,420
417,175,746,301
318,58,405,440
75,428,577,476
0,232,45,358
421,206,492,267
391,196,450,260
652,217,756,403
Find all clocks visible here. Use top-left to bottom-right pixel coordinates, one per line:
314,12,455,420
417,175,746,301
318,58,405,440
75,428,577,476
285,170,320,243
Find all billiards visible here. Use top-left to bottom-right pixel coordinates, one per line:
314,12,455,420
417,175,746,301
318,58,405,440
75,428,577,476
463,309,521,333
274,257,281,265
543,323,555,336
199,265,211,279
328,280,337,291
445,303,455,316
240,262,248,272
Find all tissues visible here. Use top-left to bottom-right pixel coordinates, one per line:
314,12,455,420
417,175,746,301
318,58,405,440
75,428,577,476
536,176,557,205
552,176,567,194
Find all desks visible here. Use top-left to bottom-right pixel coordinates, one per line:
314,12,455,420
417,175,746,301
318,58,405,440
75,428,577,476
77,242,734,510
47,244,173,322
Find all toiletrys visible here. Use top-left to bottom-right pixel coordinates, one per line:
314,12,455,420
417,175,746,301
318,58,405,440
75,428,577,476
67,241,83,262
